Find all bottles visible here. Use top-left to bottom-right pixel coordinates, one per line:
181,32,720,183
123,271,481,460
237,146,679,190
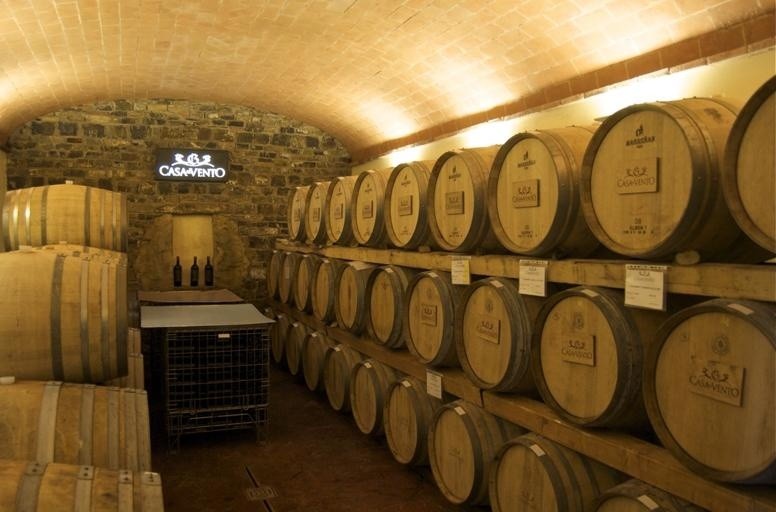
174,257,182,288
191,256,198,287
205,256,213,287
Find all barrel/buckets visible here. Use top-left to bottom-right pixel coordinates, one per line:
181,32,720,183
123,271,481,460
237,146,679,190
263,246,776,510
0,185,166,511
286,76,776,263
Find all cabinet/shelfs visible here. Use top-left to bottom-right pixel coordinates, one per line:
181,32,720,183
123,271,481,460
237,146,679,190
268,241,773,512
